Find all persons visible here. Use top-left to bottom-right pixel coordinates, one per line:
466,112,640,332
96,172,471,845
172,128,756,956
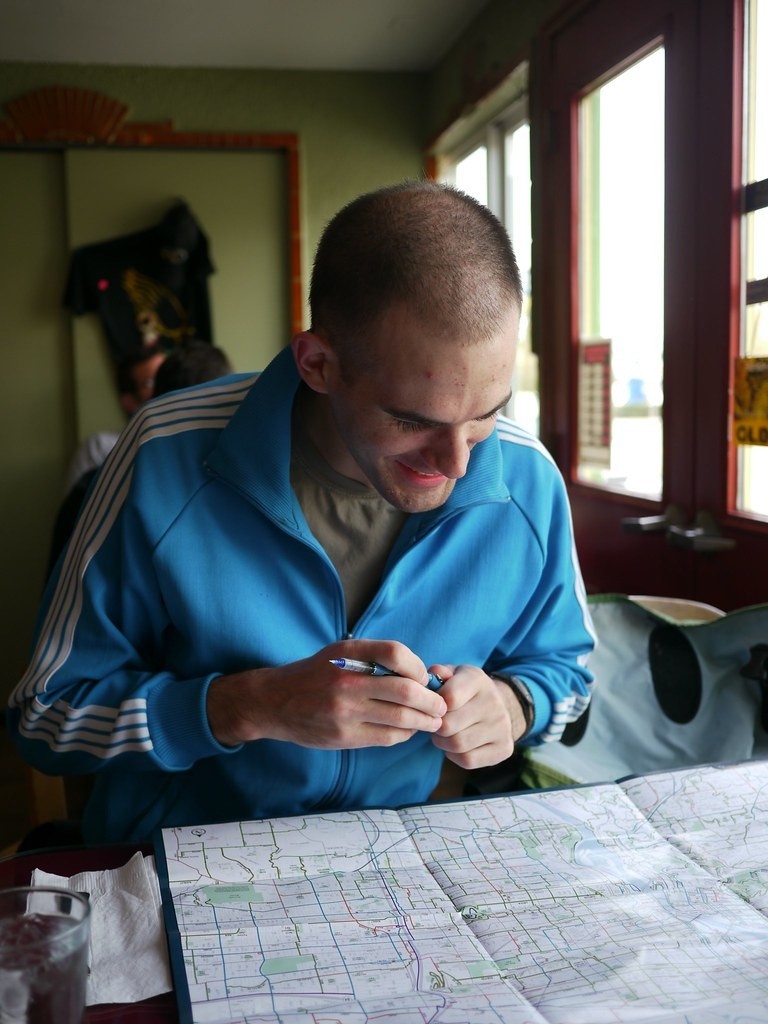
38,338,229,594
9,179,596,866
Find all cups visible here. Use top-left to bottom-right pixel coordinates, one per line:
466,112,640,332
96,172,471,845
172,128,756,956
0,886,91,1024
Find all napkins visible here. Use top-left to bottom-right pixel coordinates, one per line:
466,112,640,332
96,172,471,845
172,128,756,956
20,850,174,1007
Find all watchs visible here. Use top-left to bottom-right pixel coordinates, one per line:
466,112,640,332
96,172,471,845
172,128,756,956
490,670,535,738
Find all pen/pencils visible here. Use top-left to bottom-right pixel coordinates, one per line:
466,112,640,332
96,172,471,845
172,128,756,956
328,657,444,691
